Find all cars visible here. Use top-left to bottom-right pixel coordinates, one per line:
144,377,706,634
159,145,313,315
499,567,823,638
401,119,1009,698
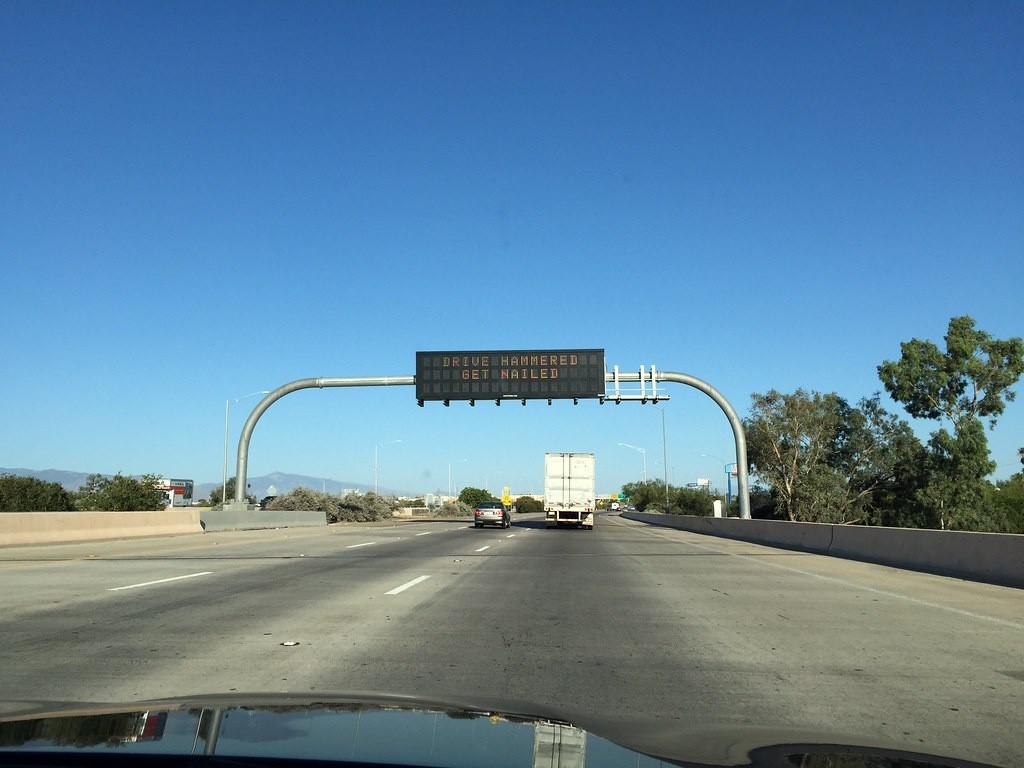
474,501,511,529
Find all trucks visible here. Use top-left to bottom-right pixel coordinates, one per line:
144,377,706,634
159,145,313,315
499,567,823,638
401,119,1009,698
544,452,595,530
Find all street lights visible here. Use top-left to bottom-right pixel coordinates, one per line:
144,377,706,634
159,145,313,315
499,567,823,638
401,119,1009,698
485,470,501,490
222,389,270,502
618,442,646,485
447,459,468,503
374,439,402,502
701,452,732,504
